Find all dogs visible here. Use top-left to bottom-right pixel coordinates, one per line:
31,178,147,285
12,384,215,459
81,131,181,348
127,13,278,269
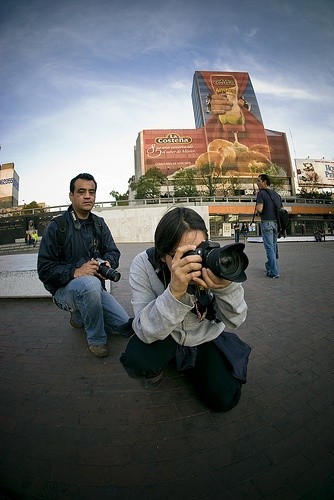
301,160,320,183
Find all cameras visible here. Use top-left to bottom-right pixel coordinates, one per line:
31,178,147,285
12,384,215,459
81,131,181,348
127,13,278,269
183,241,249,283
256,213,261,216
93,257,121,283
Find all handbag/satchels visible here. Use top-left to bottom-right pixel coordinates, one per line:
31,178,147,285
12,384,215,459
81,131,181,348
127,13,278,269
277,210,290,230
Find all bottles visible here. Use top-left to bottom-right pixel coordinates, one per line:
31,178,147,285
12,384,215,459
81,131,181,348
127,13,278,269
210,74,246,133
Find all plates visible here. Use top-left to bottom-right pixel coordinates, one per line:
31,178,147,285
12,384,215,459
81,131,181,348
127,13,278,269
166,176,288,183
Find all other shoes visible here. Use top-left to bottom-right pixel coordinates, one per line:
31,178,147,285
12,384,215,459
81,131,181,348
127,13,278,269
70,311,84,328
271,274,279,279
88,343,110,358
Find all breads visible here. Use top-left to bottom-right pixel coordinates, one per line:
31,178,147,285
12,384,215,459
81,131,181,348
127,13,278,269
195,139,271,177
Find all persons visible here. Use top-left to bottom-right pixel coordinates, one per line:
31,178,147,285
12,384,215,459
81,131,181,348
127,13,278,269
35,172,131,359
25,220,35,245
255,174,283,279
234,222,241,243
206,90,251,116
241,221,249,243
120,207,252,413
